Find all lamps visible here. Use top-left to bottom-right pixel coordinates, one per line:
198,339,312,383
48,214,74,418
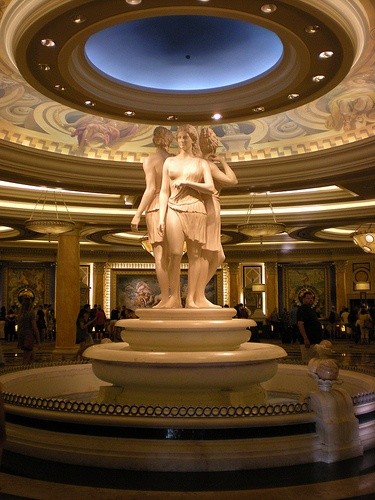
237,186,287,246
140,231,188,261
355,282,371,293
251,283,267,292
350,220,375,255
24,186,76,236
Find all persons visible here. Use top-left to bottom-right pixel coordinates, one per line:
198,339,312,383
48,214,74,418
0,292,374,364
131,126,238,308
